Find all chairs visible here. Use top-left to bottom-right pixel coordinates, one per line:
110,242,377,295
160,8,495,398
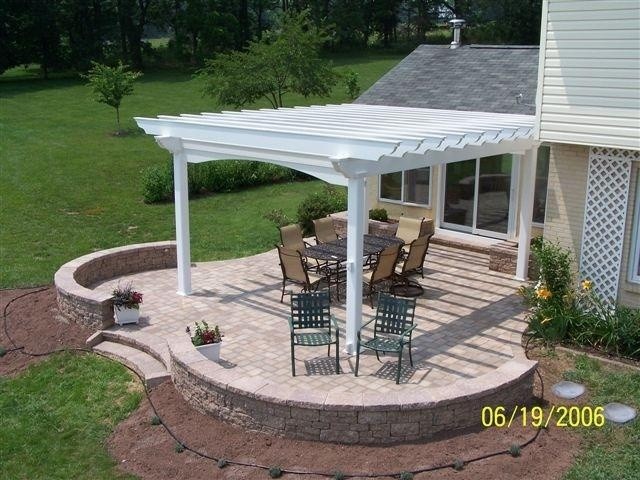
272,216,434,302
354,291,417,385
287,292,341,377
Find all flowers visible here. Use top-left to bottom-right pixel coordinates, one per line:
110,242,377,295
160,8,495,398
186,318,225,346
111,278,143,312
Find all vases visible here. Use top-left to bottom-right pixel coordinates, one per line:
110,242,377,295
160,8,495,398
114,305,141,326
195,342,221,364
